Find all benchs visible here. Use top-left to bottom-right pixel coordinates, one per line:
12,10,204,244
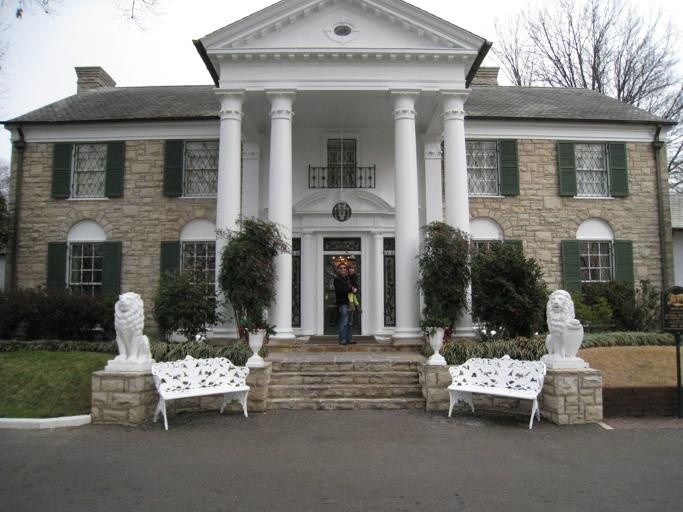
447,354,547,430
151,354,251,431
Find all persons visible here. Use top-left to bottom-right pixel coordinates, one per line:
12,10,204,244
334,263,356,346
347,263,362,313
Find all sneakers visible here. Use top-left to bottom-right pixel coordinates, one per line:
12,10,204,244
338,340,357,344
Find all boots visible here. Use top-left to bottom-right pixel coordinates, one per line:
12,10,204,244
345,303,363,313
